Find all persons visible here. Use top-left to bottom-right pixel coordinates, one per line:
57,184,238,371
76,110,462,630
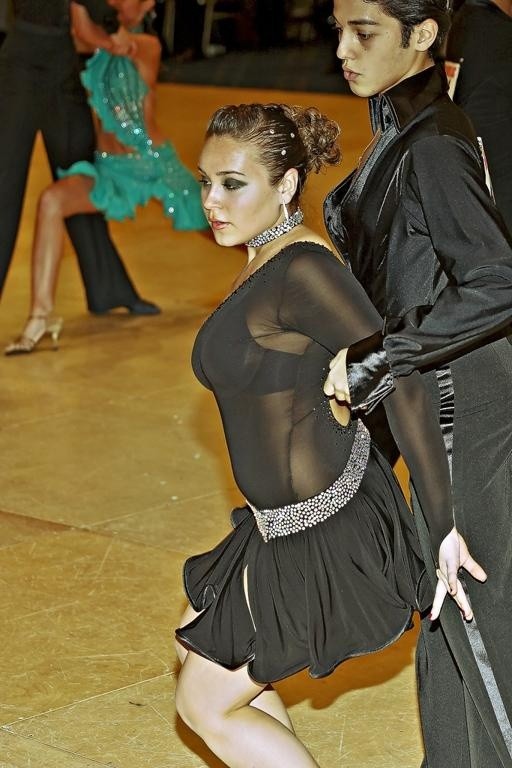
442,0,512,220
318,0,512,767
0,0,162,316
172,102,488,768
3,0,248,354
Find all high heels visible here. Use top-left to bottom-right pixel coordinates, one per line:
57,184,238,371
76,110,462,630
2,314,65,353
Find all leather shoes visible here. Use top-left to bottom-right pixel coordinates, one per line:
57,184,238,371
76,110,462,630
125,300,162,314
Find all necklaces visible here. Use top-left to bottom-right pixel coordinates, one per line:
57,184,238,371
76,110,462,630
244,206,305,248
